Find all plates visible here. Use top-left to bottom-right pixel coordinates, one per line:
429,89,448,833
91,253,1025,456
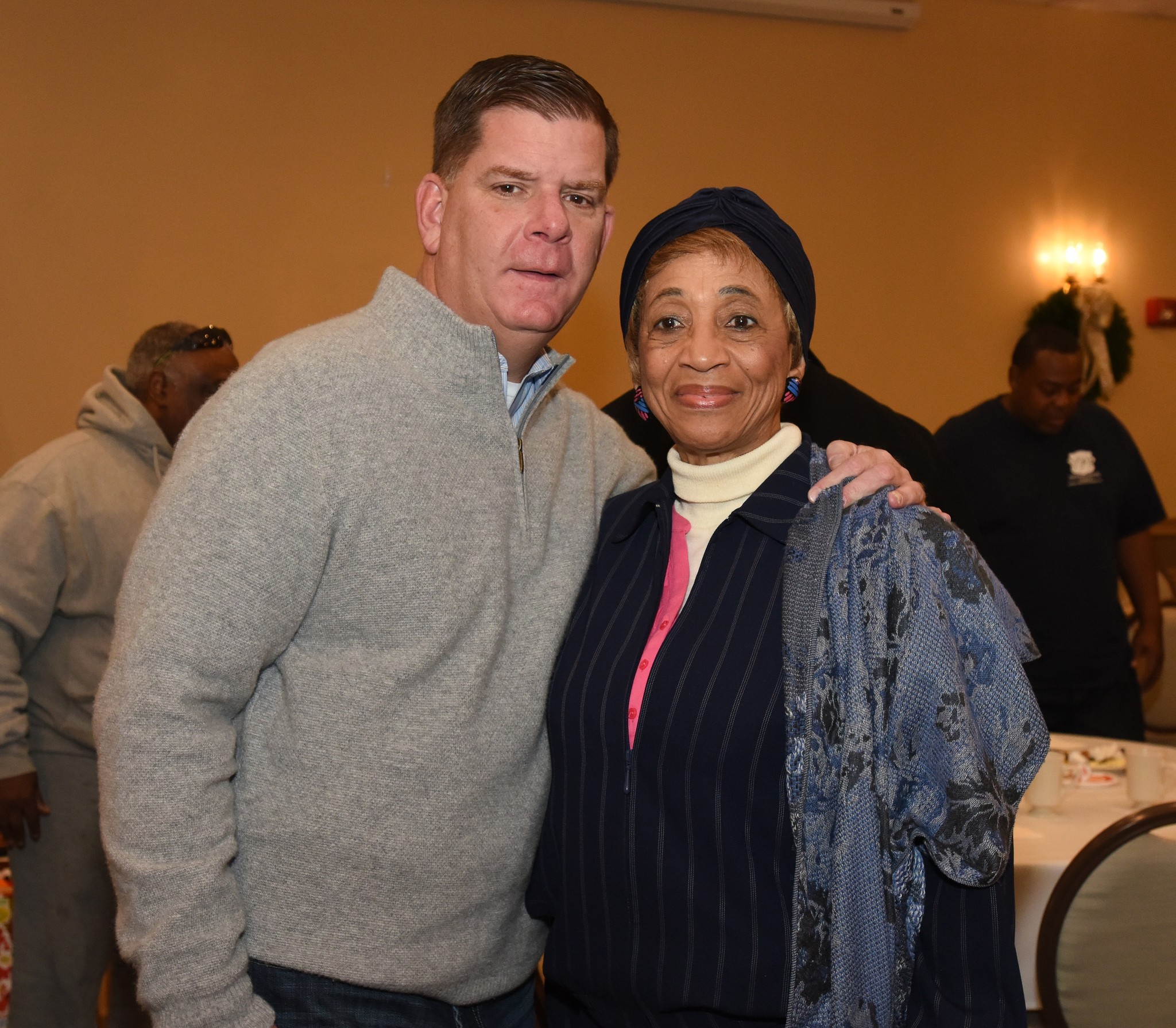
1063,772,1121,787
1064,746,1129,771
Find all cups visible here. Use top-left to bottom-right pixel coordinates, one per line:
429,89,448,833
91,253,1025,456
1023,750,1063,810
1125,748,1165,804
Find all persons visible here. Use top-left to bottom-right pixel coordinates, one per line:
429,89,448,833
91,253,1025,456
931,323,1165,743
1,322,242,1028
94,51,954,1027
525,186,1052,1028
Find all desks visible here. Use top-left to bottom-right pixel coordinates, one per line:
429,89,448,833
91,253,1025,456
1014,732,1176,1011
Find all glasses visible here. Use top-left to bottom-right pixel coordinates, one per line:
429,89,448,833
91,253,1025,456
153,325,233,372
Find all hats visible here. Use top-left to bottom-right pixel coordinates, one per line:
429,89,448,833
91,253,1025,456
620,187,816,358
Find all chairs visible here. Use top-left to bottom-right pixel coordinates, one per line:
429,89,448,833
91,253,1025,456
1035,800,1176,1028
